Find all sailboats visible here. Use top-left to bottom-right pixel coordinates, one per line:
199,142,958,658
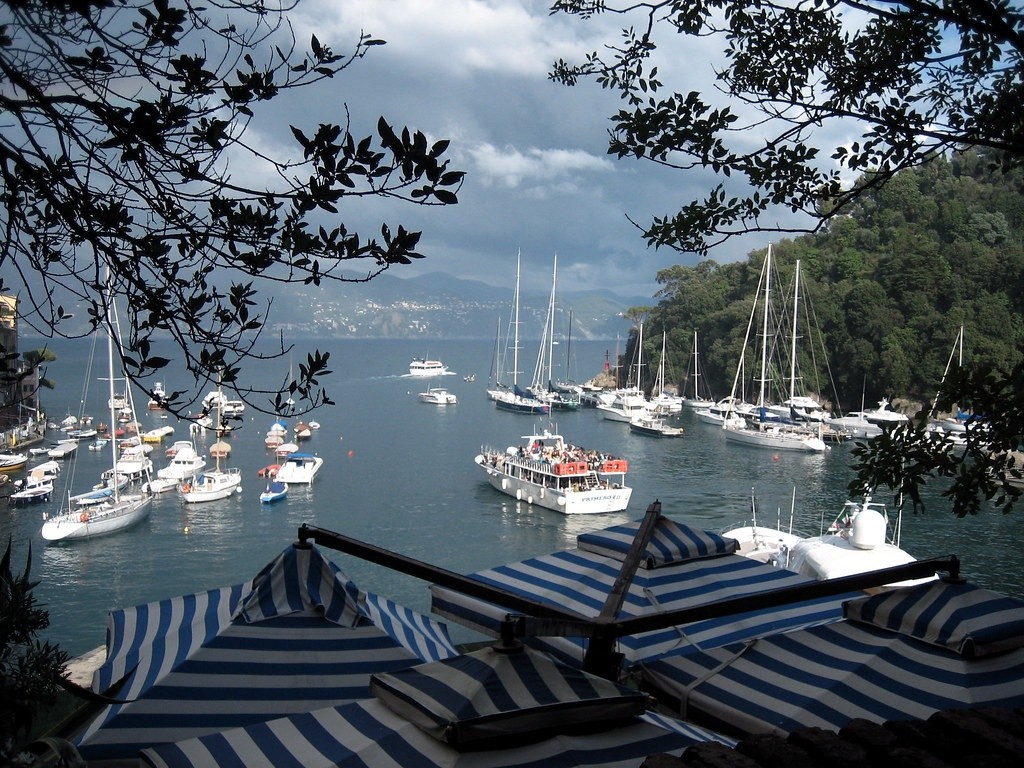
286,363,296,405
179,364,243,503
40,260,157,539
486,315,511,402
720,243,828,454
596,321,650,423
525,253,580,411
494,247,553,415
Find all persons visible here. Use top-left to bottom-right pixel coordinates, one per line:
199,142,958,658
515,437,623,491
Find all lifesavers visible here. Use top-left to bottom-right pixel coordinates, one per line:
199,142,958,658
80,513,88,522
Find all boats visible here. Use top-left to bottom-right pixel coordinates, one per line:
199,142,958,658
308,418,321,430
272,452,324,486
418,383,457,404
297,428,312,442
208,439,232,459
293,420,311,433
628,412,685,438
10,478,53,505
409,351,456,377
164,440,194,461
718,481,942,587
257,463,282,478
554,260,1006,452
141,446,207,494
474,418,634,515
259,481,289,504
0,382,245,484
266,430,285,437
272,419,288,428
100,452,153,486
275,440,300,459
268,422,287,434
69,473,131,505
264,435,285,449
